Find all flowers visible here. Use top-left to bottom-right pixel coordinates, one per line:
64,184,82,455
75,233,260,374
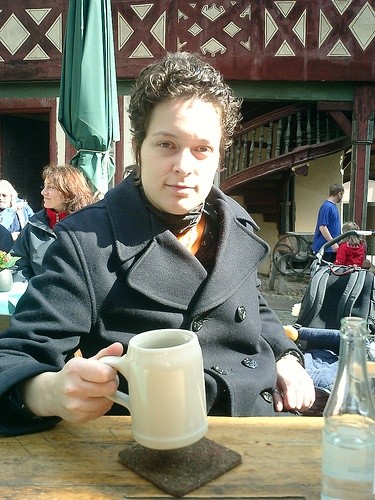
0,251,22,269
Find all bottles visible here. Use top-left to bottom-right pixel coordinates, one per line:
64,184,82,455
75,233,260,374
320,317,374,500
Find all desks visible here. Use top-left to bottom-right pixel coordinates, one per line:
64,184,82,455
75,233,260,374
0,416,375,500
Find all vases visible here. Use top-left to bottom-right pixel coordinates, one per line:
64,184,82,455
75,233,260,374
0,269,13,292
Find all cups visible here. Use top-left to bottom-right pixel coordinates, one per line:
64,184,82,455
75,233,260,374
98,329,208,450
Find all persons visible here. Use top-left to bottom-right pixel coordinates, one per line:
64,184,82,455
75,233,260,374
291,221,365,317
0,52,317,439
313,183,344,267
2,163,101,284
0,179,35,243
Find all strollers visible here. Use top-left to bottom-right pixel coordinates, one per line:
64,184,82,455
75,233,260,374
284,229,375,324
